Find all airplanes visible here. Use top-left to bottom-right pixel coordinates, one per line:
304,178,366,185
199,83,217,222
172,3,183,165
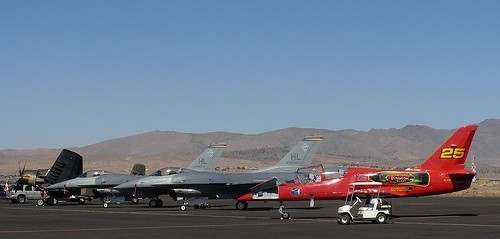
44,143,227,208
112,135,323,211
235,125,479,218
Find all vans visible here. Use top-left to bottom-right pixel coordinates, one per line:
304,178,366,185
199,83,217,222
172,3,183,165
9,183,58,205
